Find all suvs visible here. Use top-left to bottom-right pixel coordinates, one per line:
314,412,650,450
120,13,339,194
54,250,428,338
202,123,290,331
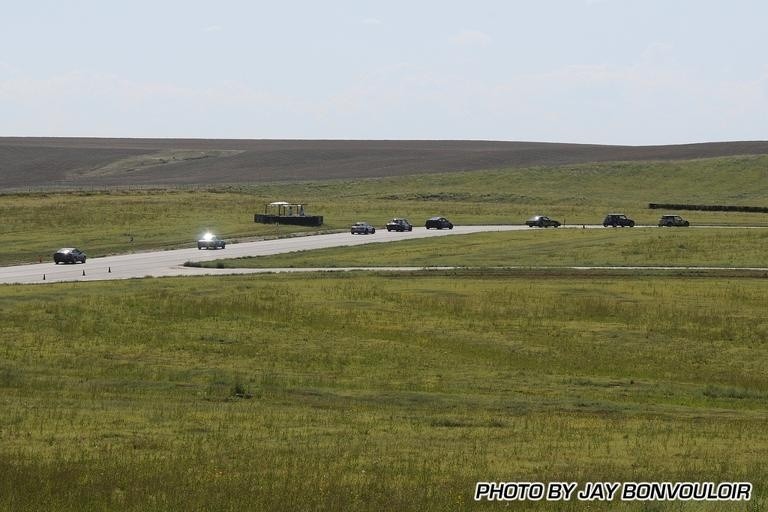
602,213,635,228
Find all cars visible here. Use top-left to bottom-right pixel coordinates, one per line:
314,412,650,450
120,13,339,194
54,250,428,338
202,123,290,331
424,217,453,229
386,218,412,232
53,248,87,264
351,222,375,234
525,215,561,228
198,233,226,250
658,215,689,227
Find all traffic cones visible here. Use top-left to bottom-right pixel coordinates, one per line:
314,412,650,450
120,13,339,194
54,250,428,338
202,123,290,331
82,270,87,276
107,266,112,273
42,273,47,281
38,256,43,264
